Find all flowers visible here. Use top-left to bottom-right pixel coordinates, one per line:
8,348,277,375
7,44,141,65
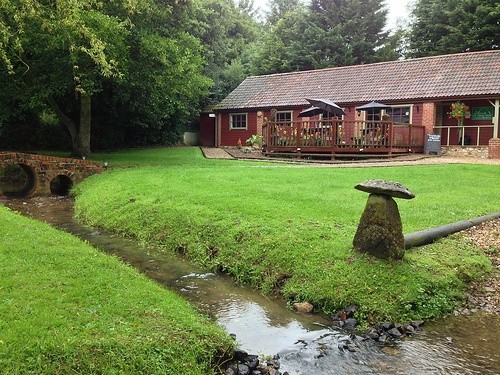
446,102,471,119
